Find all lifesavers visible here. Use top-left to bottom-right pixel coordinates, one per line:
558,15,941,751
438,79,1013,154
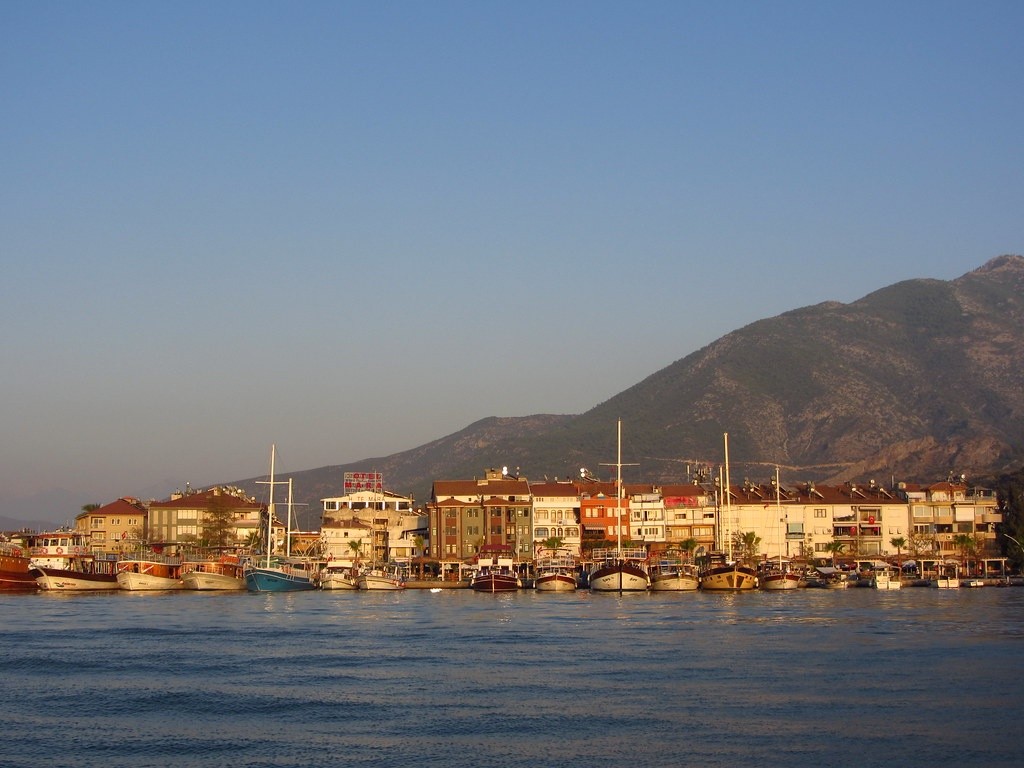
355,580,359,586
83,547,88,554
13,548,21,557
74,547,80,553
392,579,396,585
56,546,63,554
207,554,214,562
41,547,48,554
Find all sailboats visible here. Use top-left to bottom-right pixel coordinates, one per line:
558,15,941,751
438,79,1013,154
590,417,649,591
756,466,799,590
244,444,320,592
357,469,406,590
700,432,756,590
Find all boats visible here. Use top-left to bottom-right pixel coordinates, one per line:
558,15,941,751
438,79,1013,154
930,576,959,588
29,532,96,572
964,579,985,587
0,541,37,592
179,545,249,590
30,567,121,591
815,571,849,589
320,560,360,590
472,572,517,594
115,542,188,590
536,547,577,591
651,563,700,590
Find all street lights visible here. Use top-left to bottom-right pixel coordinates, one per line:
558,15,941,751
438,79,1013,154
942,526,948,560
1003,534,1024,554
933,528,942,560
